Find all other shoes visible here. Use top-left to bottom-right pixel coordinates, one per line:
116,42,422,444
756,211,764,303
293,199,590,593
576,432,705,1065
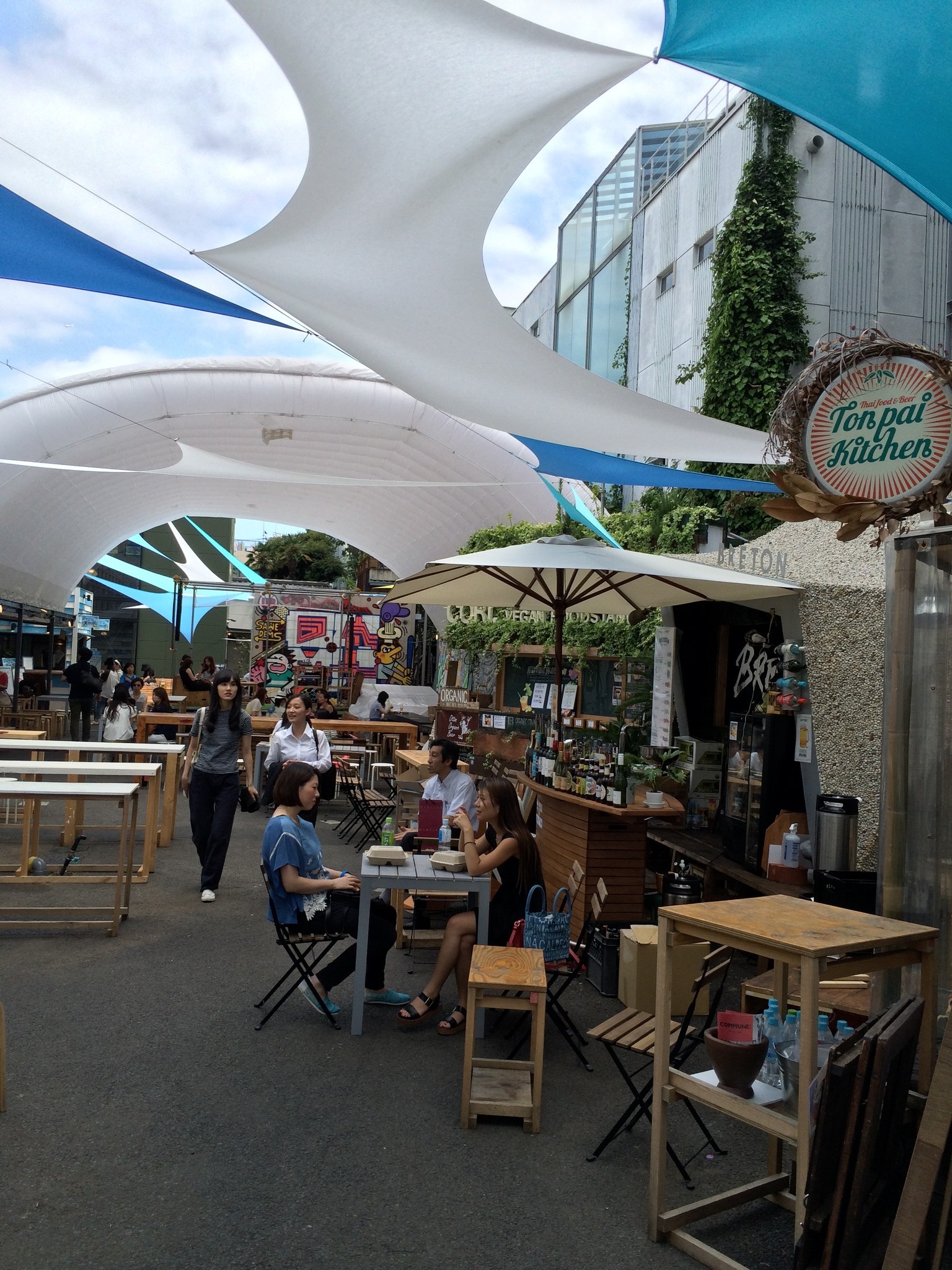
363,983,409,1008
295,975,341,1016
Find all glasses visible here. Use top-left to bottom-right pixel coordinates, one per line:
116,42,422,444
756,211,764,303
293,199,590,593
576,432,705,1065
133,683,141,687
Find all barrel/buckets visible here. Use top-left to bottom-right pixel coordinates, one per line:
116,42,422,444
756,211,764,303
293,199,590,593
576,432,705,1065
773,1039,836,1119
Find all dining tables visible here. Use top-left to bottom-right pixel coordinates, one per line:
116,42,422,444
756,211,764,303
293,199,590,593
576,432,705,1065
165,695,188,735
240,680,257,704
350,853,491,1036
134,712,418,787
1,737,186,848
0,760,162,883
0,781,141,937
384,712,433,742
0,670,73,740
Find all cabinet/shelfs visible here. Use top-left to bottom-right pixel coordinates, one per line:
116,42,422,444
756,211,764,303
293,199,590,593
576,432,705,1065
723,710,801,875
642,826,813,925
645,895,937,1270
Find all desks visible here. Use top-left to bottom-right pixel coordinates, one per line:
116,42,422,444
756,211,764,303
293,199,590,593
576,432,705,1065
395,750,470,806
739,965,868,1027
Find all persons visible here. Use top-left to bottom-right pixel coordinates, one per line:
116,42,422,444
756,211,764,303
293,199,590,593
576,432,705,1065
181,669,260,903
139,663,157,686
119,664,141,692
244,688,341,720
100,682,138,762
242,671,250,690
113,659,124,684
396,774,547,1035
107,677,148,741
143,685,178,744
179,654,221,692
369,738,488,904
724,736,764,820
260,762,411,1014
90,657,118,725
61,646,103,742
262,694,332,831
369,691,394,723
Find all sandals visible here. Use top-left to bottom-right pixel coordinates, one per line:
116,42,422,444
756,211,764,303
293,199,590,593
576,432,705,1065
438,1004,467,1036
395,989,443,1025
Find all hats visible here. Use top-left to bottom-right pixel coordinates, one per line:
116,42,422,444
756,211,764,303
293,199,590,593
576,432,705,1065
273,690,289,702
104,657,114,667
180,654,192,667
141,663,151,672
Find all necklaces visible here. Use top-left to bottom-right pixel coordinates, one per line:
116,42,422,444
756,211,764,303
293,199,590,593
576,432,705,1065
277,807,299,822
495,834,502,844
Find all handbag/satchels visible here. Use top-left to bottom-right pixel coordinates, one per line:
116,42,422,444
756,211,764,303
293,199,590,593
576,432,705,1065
183,743,200,798
148,676,155,684
520,882,572,960
312,729,336,803
511,917,524,950
78,664,103,696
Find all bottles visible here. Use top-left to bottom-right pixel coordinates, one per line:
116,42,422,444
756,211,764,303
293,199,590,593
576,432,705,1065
525,714,627,808
381,818,395,846
761,997,854,1090
438,819,451,851
783,823,800,869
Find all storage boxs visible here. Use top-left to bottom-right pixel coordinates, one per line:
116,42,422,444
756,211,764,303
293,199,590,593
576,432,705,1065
673,735,724,830
394,764,437,813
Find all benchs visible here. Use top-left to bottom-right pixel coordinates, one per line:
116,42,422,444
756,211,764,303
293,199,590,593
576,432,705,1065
171,673,211,710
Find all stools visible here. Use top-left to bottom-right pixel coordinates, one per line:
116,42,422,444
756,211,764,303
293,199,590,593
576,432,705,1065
459,944,549,1134
370,763,395,800
150,733,426,801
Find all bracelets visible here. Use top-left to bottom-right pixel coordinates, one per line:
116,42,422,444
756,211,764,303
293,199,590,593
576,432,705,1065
463,841,477,847
340,870,352,877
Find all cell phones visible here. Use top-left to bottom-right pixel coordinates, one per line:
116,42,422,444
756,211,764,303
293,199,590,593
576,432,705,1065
201,663,205,669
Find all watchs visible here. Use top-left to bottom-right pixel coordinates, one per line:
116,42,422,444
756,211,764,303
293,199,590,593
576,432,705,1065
144,706,147,709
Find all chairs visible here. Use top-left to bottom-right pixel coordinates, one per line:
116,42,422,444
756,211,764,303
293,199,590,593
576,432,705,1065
253,855,350,1030
504,878,608,1071
378,772,397,815
402,835,468,974
556,859,585,915
331,756,401,854
491,758,519,790
578,945,736,1190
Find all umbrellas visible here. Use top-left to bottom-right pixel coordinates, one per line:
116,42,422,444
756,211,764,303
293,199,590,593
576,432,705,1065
377,537,805,742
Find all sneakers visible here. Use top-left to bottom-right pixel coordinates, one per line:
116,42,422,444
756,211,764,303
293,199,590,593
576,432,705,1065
200,888,217,903
